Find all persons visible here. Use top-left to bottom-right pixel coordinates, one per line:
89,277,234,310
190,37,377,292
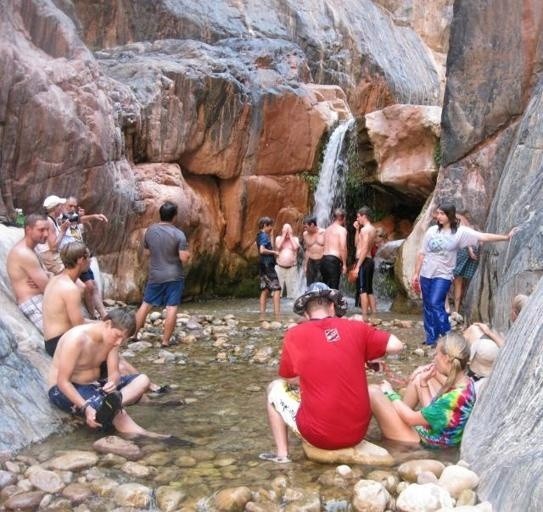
134,202,190,347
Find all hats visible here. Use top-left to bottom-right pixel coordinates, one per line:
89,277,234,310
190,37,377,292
467,339,500,377
293,281,348,317
43,195,66,211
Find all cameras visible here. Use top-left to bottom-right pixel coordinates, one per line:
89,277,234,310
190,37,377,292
365,362,383,372
62,212,79,224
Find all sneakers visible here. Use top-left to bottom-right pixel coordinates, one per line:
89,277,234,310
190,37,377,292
259,453,292,464
95,391,122,425
